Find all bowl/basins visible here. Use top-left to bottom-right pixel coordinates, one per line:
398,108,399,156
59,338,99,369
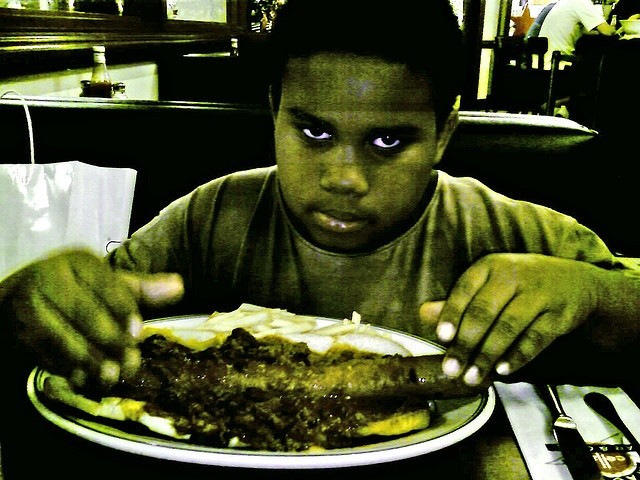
618,18,639,34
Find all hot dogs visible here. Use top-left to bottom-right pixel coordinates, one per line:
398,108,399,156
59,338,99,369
43,310,482,453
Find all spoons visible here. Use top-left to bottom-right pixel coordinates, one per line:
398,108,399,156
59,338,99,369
583,392,640,453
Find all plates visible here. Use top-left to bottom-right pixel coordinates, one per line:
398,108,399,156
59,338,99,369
25,314,497,470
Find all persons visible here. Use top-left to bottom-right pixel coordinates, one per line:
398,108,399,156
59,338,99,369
607,1,640,37
527,2,556,37
531,1,614,119
0,0,640,392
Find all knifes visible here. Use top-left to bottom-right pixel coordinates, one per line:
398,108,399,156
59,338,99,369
532,373,604,480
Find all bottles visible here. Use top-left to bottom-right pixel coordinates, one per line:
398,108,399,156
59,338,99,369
91,46,110,97
609,14,618,26
